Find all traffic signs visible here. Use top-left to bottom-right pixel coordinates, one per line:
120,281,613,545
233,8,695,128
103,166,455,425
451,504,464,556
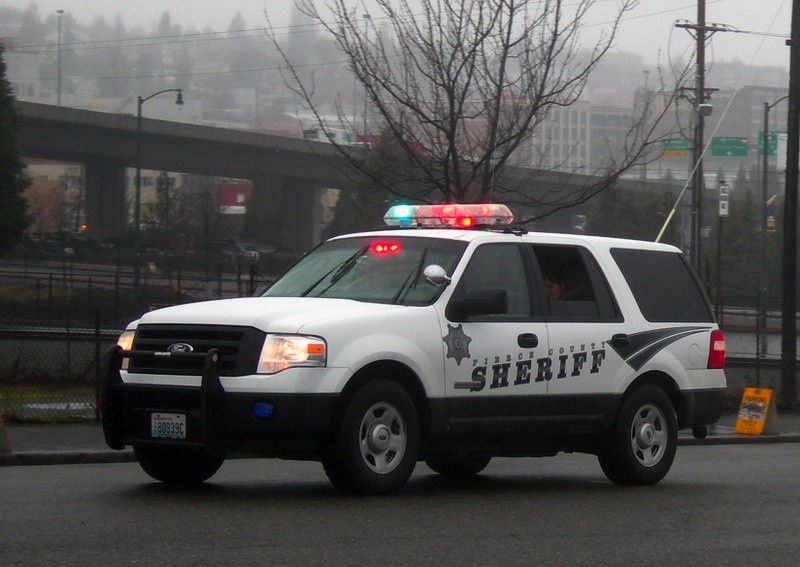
757,130,788,158
660,137,690,158
710,136,749,158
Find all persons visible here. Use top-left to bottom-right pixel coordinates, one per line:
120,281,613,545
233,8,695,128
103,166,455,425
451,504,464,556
541,261,594,301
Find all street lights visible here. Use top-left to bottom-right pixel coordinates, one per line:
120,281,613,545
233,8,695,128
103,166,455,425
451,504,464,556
692,102,713,281
131,87,185,320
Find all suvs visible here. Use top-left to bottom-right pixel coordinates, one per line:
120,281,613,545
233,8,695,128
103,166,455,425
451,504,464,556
96,199,730,499
